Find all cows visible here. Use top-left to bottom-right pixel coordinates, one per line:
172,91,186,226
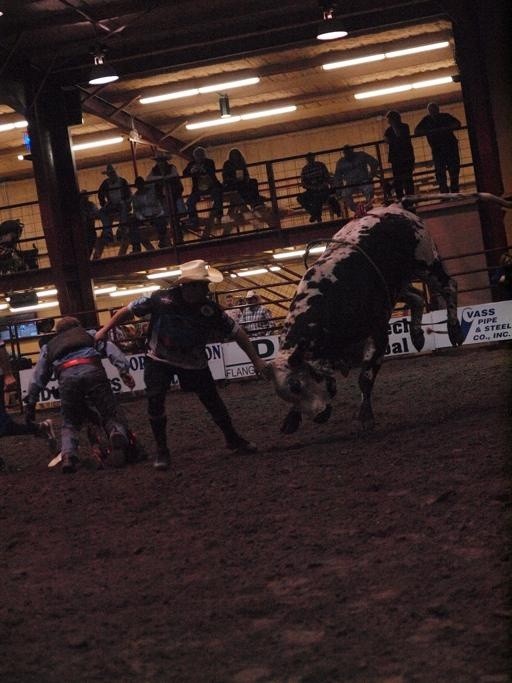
259,188,511,430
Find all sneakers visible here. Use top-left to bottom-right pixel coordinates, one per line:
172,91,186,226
147,415,170,470
212,414,258,455
109,426,128,468
61,453,74,473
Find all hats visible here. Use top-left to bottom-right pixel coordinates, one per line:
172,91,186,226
100,163,117,175
169,259,224,284
149,151,170,161
244,290,260,300
80,189,91,197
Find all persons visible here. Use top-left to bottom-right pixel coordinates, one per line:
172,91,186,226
239,290,276,338
109,310,151,357
224,292,243,325
23,316,137,473
78,143,267,257
331,144,381,213
0,337,58,452
382,105,417,204
93,259,272,472
414,105,462,202
296,151,330,226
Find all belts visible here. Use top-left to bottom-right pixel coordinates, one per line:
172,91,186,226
57,357,102,375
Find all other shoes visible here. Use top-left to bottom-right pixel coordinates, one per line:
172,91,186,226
253,194,273,214
438,192,462,201
102,226,184,255
209,208,227,223
309,210,323,223
398,196,414,209
349,202,373,212
187,212,198,227
